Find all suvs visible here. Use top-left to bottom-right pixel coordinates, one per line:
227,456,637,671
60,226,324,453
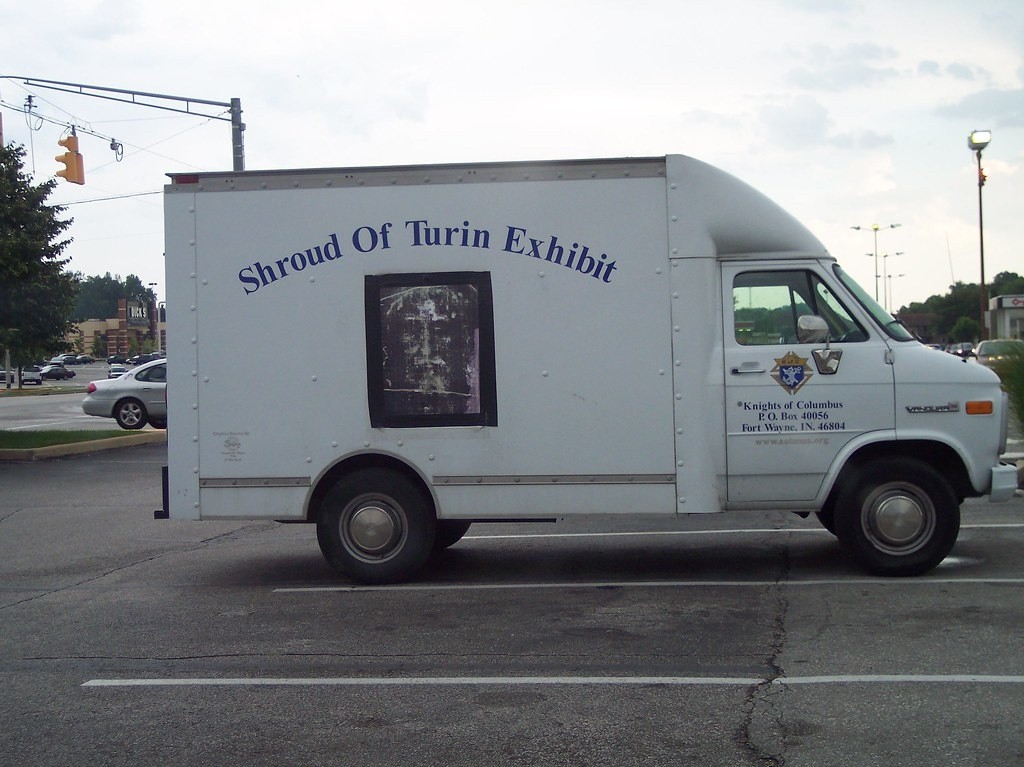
21,365,42,384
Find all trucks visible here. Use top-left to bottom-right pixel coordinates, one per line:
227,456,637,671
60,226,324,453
152,154,1022,589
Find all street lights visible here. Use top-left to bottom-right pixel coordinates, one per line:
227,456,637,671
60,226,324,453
965,127,992,345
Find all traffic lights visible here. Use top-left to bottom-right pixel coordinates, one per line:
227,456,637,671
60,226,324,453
56,135,85,186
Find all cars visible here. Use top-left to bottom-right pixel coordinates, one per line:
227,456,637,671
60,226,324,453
38,367,77,380
38,354,96,370
108,366,128,380
0,367,15,383
82,356,168,432
106,350,166,366
927,340,980,358
